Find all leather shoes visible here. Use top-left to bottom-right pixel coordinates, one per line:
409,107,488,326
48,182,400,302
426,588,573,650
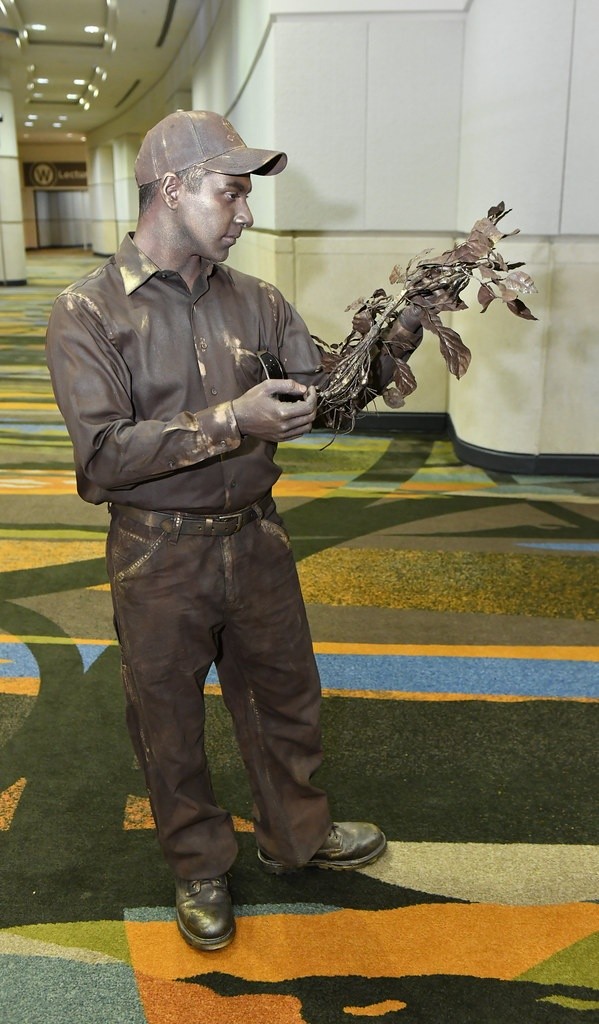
257,821,388,871
175,870,236,952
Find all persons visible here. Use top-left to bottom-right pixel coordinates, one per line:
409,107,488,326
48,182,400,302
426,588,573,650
44,110,471,949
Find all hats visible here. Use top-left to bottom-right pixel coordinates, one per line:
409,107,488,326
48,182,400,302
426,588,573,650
134,108,288,187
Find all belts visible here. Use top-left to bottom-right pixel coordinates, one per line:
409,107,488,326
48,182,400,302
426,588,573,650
110,500,277,536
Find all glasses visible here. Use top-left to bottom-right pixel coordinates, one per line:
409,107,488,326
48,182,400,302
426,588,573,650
258,350,285,396
